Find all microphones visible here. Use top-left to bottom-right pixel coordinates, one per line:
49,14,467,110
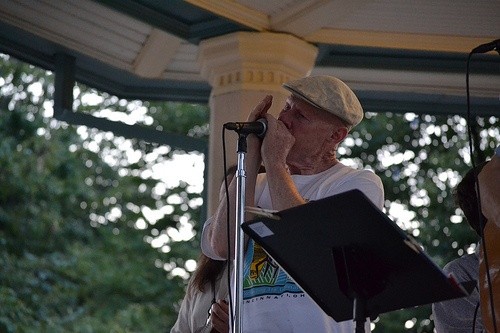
472,39,500,54
225,119,268,138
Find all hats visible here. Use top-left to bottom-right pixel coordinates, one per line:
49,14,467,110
282,75,364,126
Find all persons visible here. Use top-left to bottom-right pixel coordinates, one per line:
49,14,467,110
432,159,489,333
168,164,266,333
475,143,500,229
201,75,384,333
